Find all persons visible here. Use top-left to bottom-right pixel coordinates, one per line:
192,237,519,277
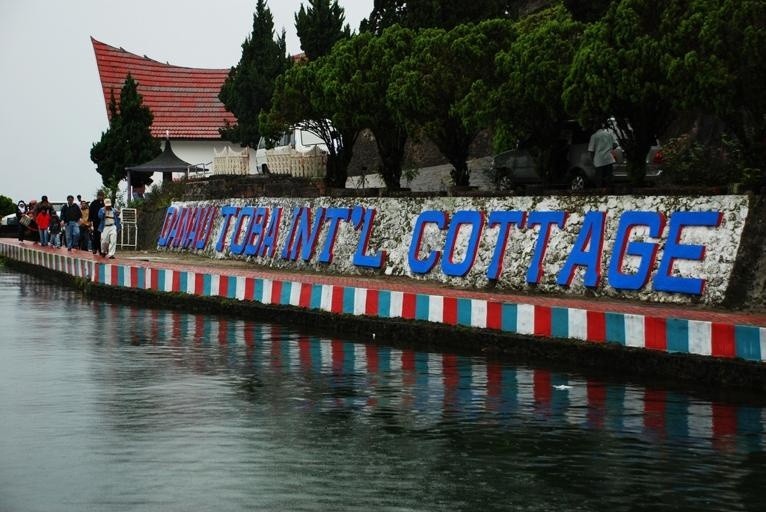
16,191,121,259
587,120,619,188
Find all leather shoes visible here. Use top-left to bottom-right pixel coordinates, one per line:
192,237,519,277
102,253,107,258
109,255,116,259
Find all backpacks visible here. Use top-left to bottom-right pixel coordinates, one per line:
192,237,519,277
51,219,60,234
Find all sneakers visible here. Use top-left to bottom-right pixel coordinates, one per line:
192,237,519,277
17,241,97,254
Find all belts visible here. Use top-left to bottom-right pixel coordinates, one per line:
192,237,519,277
105,223,116,226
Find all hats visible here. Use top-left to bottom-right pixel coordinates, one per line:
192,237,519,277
104,198,112,207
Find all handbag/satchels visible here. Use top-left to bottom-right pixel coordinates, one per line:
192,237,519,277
19,214,31,227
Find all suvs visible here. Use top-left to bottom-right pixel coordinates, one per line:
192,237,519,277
485,119,668,193
0,200,68,226
